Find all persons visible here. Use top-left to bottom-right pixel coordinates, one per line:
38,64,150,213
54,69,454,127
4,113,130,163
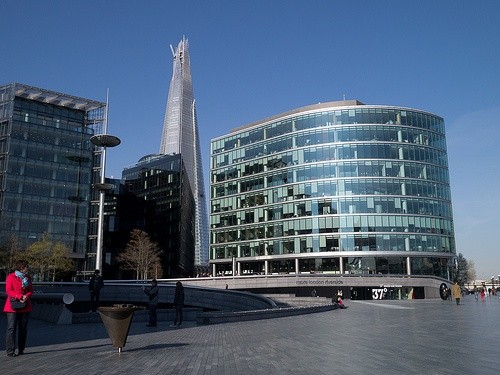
88,270,105,314
3,260,33,357
443,281,497,305
169,281,184,329
332,293,348,308
141,279,160,327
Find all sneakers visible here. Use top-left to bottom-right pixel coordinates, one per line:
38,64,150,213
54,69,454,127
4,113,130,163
89,310,184,329
18,351,26,355
7,352,18,357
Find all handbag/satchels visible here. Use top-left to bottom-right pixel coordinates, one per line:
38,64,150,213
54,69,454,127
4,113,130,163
11,298,28,310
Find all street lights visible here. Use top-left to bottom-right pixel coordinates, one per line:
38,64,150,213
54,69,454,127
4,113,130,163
492,276,495,288
64,155,91,254
497,275,500,283
89,134,122,281
491,278,493,289
495,279,498,284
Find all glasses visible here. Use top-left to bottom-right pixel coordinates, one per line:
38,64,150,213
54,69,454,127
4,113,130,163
23,265,28,268
